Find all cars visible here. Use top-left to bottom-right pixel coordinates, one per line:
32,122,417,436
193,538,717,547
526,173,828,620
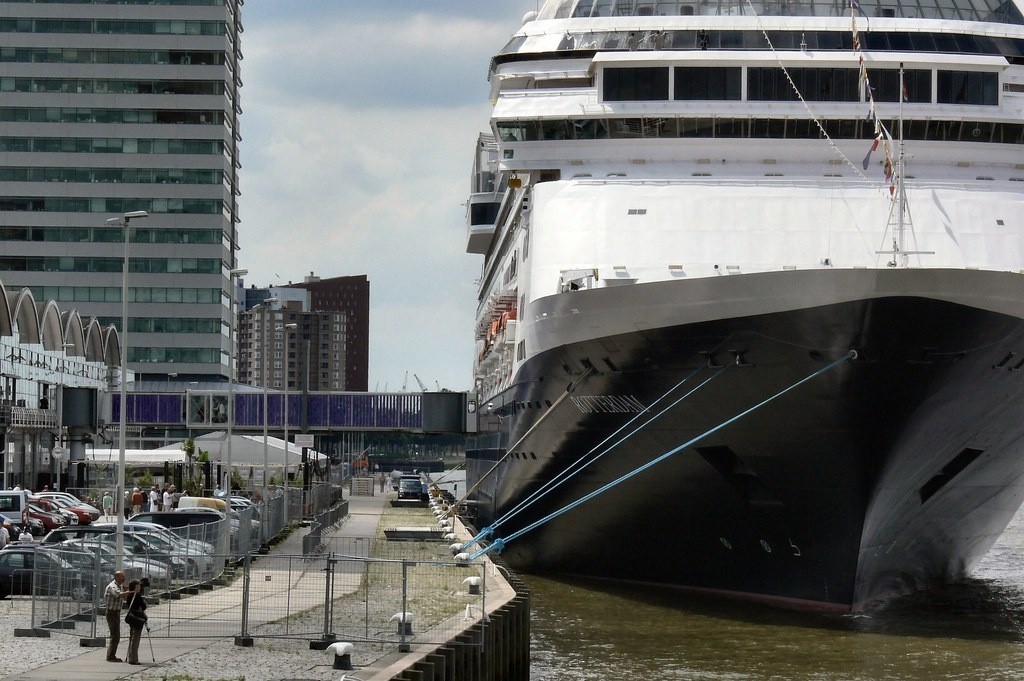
0,492,214,598
398,479,423,500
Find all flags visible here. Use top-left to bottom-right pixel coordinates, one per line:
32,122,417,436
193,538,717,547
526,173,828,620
903,81,908,101
851,11,897,196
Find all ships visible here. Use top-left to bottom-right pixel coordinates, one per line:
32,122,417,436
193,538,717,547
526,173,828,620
454,0,1024,615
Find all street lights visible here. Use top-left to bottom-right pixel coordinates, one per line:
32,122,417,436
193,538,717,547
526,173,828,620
283,323,297,525
118,210,151,590
261,299,279,549
225,269,251,565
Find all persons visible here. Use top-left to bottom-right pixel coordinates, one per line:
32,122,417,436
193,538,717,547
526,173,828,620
212,401,225,419
124,580,148,665
104,571,129,662
139,488,148,512
124,490,131,520
18,526,34,543
6,481,58,495
132,487,143,514
379,472,385,493
40,396,48,409
103,491,113,522
213,485,221,498
0,520,10,550
385,474,393,493
148,483,187,512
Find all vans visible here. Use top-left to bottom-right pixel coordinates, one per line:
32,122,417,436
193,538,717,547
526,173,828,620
178,498,237,514
0,489,30,532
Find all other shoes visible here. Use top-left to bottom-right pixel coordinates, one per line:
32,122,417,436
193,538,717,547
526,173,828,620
106,656,122,662
129,662,140,665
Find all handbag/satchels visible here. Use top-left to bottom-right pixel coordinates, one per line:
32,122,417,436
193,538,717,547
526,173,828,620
154,500,158,505
125,611,148,629
173,502,178,508
